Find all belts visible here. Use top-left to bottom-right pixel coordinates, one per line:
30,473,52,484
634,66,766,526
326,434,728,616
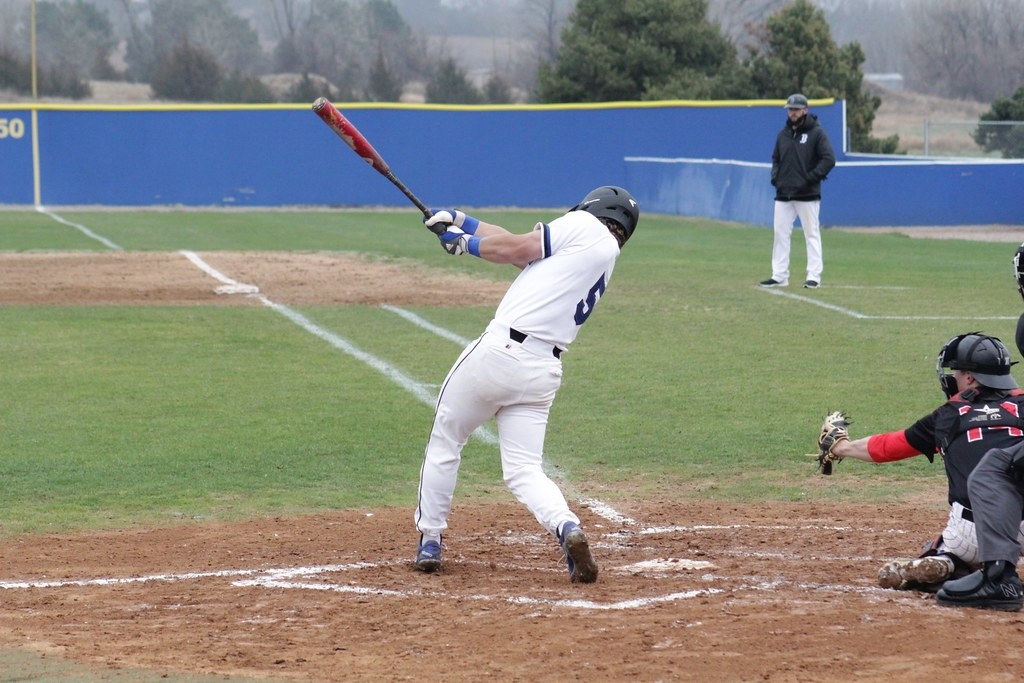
962,507,976,523
509,327,562,360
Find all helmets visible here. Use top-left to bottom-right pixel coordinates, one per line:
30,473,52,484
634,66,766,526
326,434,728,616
947,334,1019,389
1012,243,1024,298
569,186,640,240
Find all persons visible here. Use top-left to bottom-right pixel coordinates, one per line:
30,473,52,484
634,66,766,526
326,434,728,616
935,242,1024,611
414,185,639,583
760,94,835,288
816,332,1024,592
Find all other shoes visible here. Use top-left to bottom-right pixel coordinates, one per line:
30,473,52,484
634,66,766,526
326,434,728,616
935,567,1024,612
876,555,955,588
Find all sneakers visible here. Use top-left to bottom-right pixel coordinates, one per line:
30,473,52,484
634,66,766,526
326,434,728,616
804,279,818,288
418,533,443,570
555,521,598,582
758,278,790,288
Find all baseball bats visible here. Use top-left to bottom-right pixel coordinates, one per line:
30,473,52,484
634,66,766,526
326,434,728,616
311,96,447,235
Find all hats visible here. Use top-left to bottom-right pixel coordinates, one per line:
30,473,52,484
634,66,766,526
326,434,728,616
783,94,808,109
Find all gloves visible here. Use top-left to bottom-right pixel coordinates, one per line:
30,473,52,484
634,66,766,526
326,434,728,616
438,224,482,259
422,207,480,237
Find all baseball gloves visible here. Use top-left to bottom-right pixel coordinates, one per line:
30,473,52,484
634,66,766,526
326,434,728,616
817,410,852,476
